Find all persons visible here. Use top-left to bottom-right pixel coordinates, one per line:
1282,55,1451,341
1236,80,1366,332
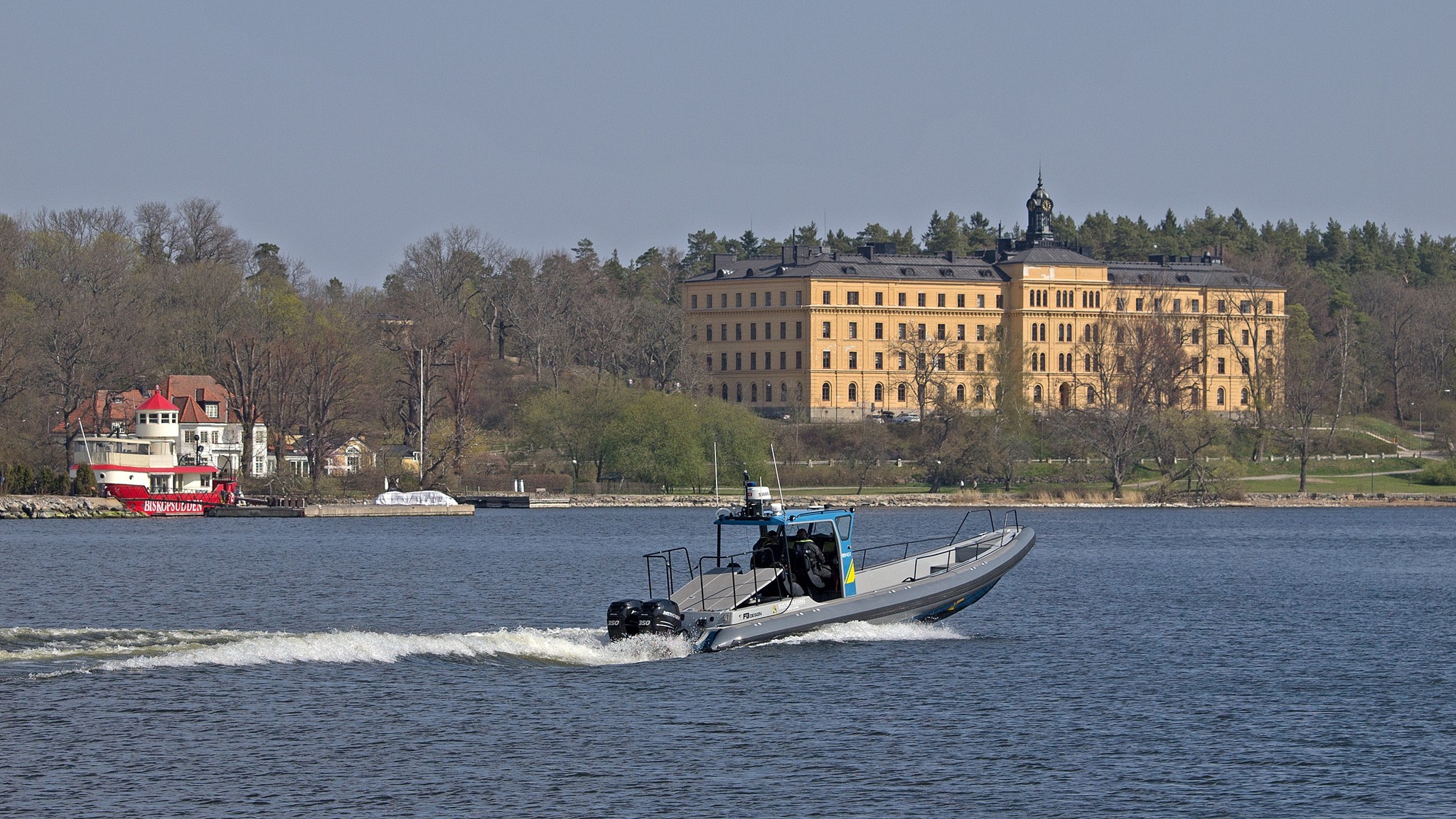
792,528,834,577
147,483,166,493
750,530,780,570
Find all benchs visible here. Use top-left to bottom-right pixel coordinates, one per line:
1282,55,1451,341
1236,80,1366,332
536,488,565,497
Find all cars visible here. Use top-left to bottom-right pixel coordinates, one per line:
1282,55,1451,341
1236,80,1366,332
891,412,921,424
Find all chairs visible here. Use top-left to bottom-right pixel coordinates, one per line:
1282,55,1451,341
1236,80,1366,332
728,544,825,601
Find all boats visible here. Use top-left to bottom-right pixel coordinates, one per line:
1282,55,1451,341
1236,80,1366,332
68,385,238,518
605,443,1041,657
733,470,788,517
